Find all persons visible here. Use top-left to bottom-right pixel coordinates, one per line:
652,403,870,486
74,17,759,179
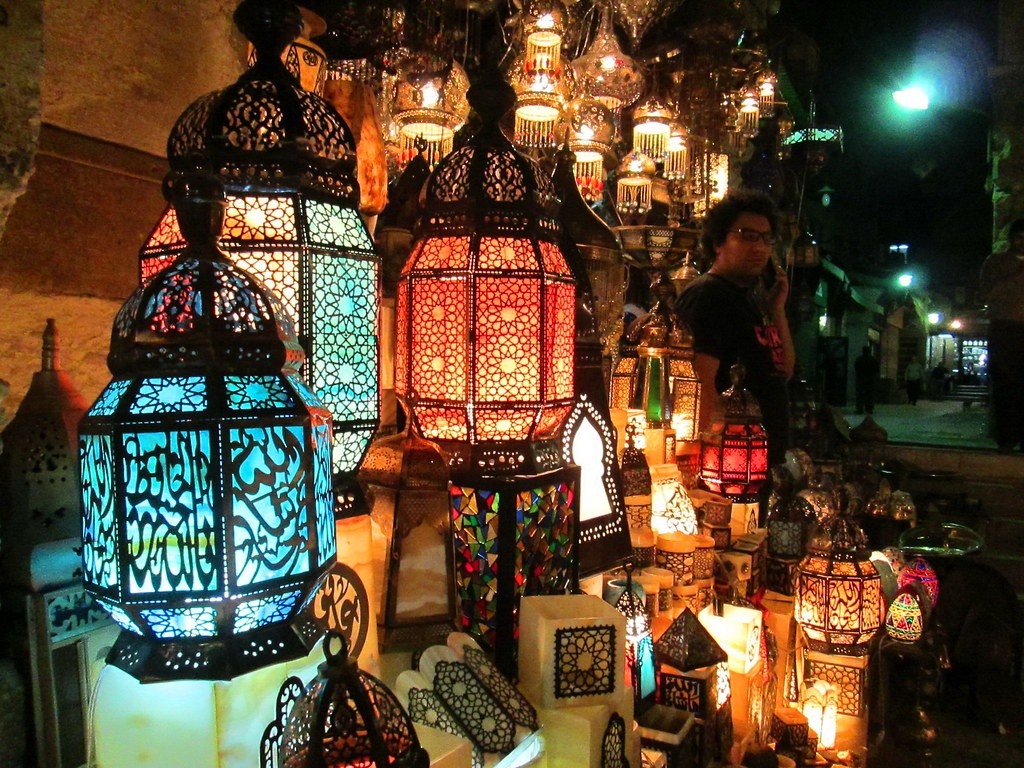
902,355,926,405
852,346,881,416
674,188,797,468
930,360,951,401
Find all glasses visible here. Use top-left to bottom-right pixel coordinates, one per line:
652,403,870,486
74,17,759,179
729,228,774,243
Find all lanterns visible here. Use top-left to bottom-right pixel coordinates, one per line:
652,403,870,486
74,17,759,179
0,0,773,768
678,418,999,767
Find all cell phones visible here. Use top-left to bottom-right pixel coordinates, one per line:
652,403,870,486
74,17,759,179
761,256,778,292
